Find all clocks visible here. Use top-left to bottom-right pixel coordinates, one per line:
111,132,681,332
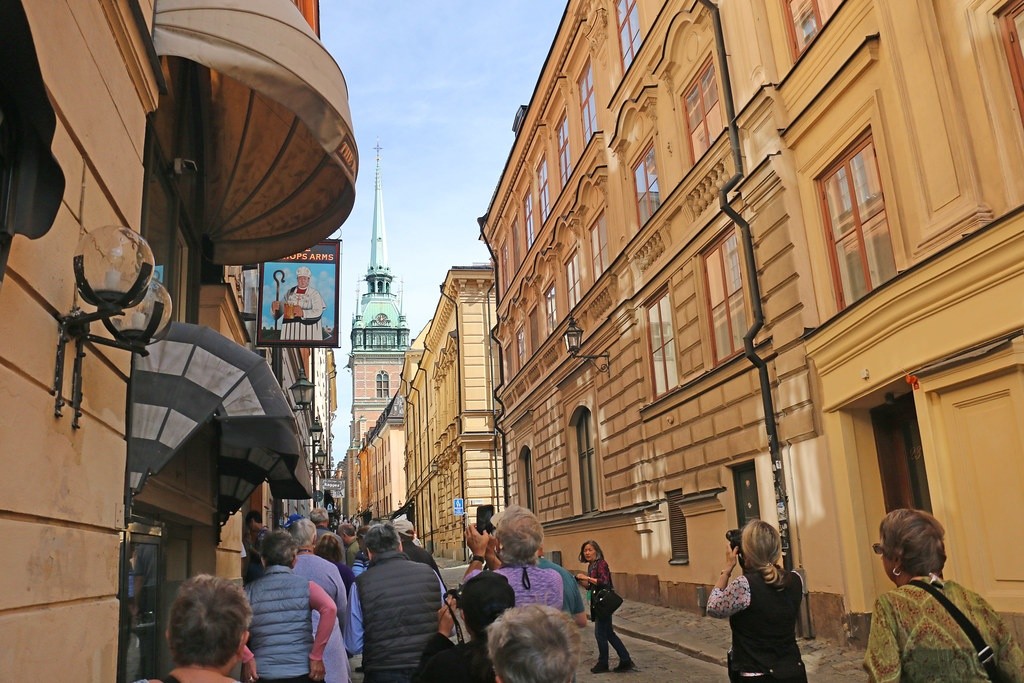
376,314,387,325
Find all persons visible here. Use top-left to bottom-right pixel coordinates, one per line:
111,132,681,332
132,500,636,683
861,508,1024,683
706,518,810,683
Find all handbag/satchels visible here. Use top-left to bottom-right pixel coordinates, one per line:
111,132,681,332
590,587,623,620
243,551,266,584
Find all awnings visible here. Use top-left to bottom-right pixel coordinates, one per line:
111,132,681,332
124,320,314,520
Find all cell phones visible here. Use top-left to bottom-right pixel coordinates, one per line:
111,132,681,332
477,505,494,535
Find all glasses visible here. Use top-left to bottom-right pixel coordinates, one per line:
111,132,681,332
872,542,889,555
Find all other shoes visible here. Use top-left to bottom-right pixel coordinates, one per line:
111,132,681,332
591,663,609,672
614,660,635,671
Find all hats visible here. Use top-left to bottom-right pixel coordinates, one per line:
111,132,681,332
456,570,515,618
283,513,305,528
490,511,506,529
393,519,415,536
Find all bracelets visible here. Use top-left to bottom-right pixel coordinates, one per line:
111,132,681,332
721,569,731,577
472,555,485,564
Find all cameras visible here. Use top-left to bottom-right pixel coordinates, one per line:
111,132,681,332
451,584,464,608
726,529,742,552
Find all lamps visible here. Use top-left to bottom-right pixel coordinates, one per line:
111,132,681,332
562,313,611,379
310,448,327,471
288,368,315,415
303,418,324,449
53,226,173,431
